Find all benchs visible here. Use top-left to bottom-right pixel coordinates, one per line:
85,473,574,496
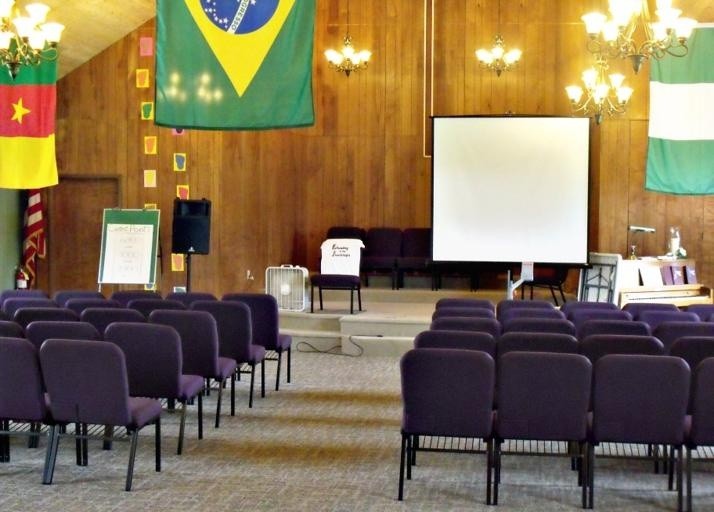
620,284,713,310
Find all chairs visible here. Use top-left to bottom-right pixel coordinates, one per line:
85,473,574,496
309,226,570,314
396,298,714,512
0,289,292,491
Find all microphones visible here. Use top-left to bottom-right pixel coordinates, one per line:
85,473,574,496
627,226,656,235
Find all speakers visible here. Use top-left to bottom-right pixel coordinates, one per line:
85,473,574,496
170,199,211,255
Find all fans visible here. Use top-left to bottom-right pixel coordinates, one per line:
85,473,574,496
265,264,309,313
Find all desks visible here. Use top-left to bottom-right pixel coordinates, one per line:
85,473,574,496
613,256,697,307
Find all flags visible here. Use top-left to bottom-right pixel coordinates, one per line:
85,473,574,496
22,189,47,289
643,23,714,196
0,40,59,190
154,0,316,129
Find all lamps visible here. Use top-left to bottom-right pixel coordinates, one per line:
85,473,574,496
580,0,698,74
324,0,372,77
0,0,65,82
475,0,523,76
565,63,634,124
